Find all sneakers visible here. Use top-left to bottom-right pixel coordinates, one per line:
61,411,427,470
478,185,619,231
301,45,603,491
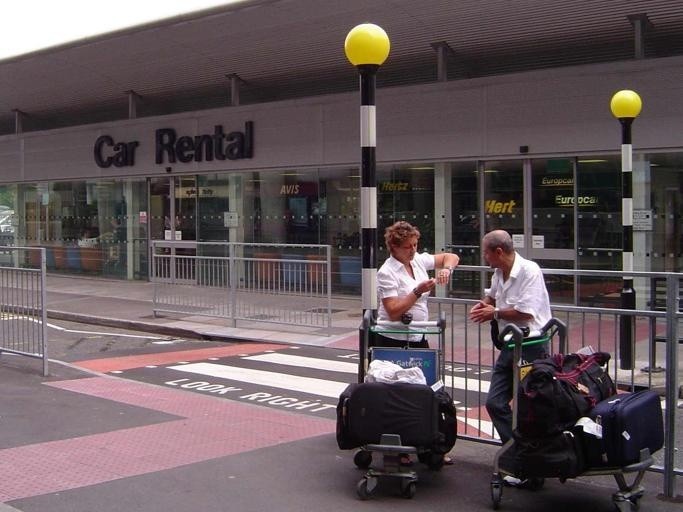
503,474,528,486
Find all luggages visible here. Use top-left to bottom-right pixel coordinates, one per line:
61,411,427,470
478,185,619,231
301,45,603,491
581,387,665,472
335,380,458,456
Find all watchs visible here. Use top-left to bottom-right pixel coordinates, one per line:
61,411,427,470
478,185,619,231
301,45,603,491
494,308,501,321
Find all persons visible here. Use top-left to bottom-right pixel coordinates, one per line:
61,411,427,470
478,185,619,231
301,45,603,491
77,230,100,249
471,229,552,489
374,221,460,467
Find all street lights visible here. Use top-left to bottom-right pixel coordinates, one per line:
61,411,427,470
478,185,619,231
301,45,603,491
338,23,392,384
609,85,640,373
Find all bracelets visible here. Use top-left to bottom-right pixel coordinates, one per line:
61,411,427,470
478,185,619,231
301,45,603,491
444,265,454,274
412,289,422,299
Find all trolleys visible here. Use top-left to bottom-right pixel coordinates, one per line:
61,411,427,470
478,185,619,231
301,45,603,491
338,309,452,498
480,313,656,511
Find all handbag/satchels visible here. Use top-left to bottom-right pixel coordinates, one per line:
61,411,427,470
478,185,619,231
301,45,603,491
510,347,622,444
498,440,579,479
